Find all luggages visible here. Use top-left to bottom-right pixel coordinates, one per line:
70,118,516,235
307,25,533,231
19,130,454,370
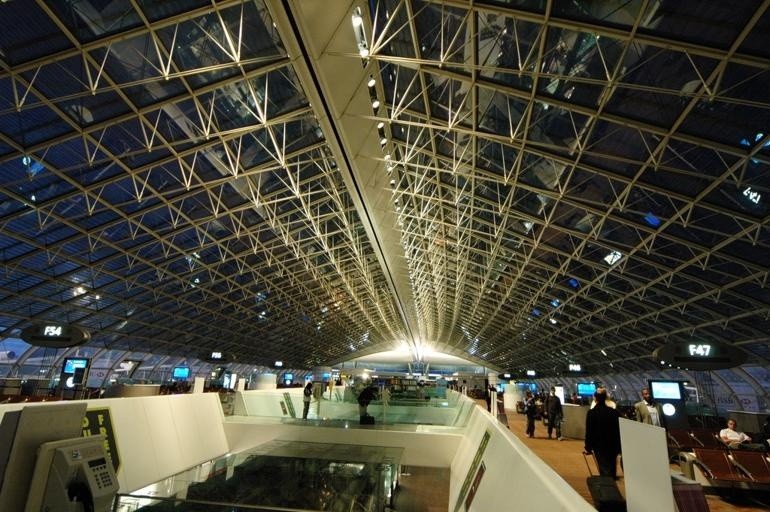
582,450,628,512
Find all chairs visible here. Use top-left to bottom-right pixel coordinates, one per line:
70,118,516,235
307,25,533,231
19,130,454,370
664,428,770,512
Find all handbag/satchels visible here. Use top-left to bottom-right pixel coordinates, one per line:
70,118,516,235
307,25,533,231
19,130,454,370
360,413,375,425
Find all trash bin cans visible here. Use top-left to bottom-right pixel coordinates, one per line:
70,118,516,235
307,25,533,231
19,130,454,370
516,400,525,413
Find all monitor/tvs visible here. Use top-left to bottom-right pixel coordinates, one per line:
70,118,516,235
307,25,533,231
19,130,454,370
304,375,312,381
322,372,330,378
63,359,88,374
651,382,681,400
284,373,294,380
515,382,525,386
173,367,190,378
577,383,598,396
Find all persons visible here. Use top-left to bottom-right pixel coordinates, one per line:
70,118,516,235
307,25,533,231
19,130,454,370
634,389,665,429
524,387,564,440
302,383,313,420
585,387,622,482
485,383,497,412
718,419,769,453
357,386,379,421
